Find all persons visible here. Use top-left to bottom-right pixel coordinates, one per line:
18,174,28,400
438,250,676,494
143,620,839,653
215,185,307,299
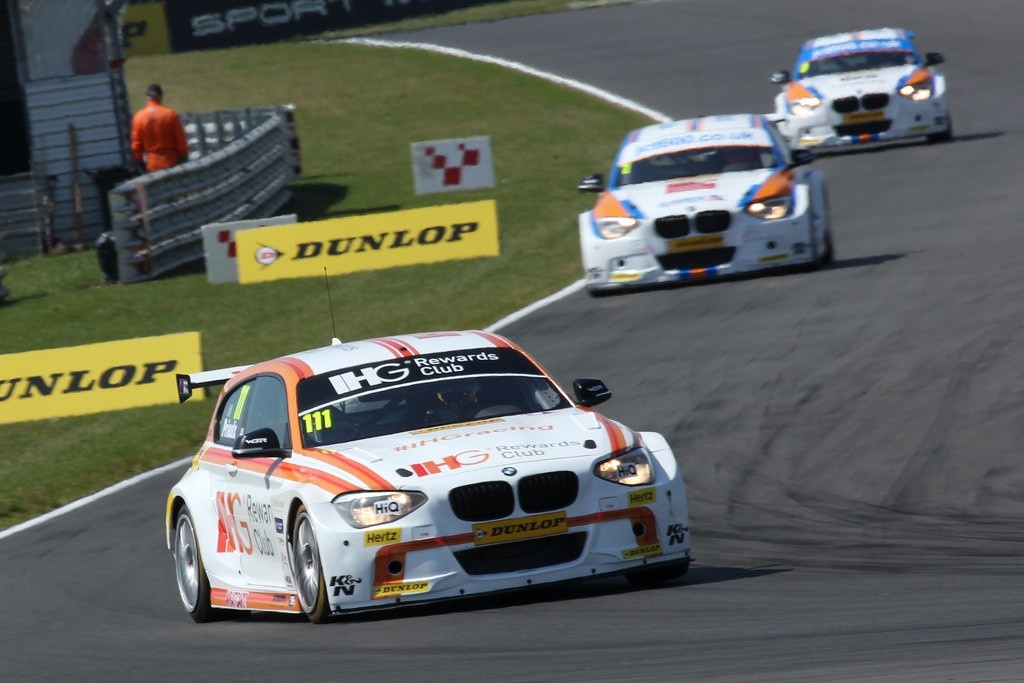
129,82,190,172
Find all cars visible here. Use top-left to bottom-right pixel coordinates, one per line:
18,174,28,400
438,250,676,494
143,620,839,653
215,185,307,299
770,26,953,156
164,267,694,621
576,114,830,296
